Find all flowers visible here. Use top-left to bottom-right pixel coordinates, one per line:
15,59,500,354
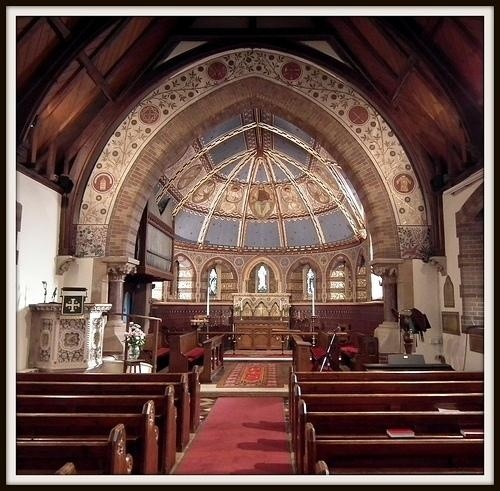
122,322,145,356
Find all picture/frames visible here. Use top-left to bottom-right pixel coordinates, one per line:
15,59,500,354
0,0,500,491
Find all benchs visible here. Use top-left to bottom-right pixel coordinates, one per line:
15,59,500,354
143,330,205,374
15,366,202,476
288,366,482,474
312,330,379,372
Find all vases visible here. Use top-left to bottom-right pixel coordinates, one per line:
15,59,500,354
128,344,140,360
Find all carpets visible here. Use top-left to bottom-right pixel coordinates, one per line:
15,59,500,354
216,361,283,388
173,398,293,476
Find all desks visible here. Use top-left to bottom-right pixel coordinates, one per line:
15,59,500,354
122,359,144,373
